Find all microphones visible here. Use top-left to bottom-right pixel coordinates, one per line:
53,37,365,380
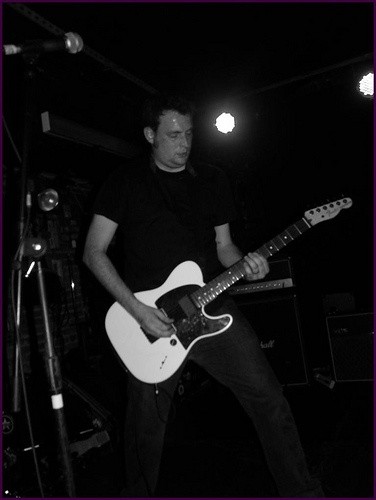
2,32,84,56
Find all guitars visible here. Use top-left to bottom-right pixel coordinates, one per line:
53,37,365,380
104,196,353,385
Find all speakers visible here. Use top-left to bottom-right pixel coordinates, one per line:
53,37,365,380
232,294,309,387
325,310,374,383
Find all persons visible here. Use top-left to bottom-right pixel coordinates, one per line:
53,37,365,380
80,93,324,498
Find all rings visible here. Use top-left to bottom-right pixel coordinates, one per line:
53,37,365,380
253,270,259,274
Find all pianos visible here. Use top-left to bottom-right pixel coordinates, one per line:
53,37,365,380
208,256,294,300
